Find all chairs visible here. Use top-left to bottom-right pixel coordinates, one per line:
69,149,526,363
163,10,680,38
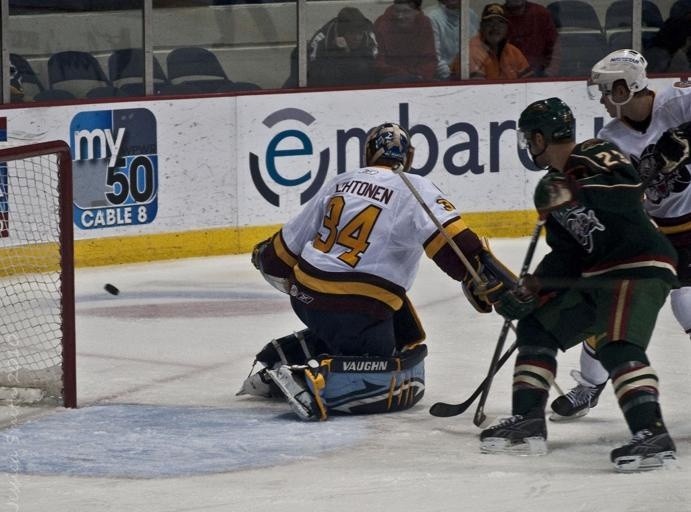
9,45,261,104
548,0,663,76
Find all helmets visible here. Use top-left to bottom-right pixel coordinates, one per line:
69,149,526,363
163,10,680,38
363,122,410,165
517,100,575,172
588,48,649,108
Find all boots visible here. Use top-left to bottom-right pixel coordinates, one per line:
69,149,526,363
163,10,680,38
552,371,607,416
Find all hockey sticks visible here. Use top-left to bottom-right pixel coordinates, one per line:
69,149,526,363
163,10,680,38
429,339,521,417
473,219,542,429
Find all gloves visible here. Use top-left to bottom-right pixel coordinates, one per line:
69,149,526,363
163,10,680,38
497,290,544,319
537,177,574,209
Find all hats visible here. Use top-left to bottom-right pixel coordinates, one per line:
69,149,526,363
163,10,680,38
483,4,508,24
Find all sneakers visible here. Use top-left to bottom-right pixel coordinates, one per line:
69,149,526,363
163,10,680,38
610,420,676,462
242,364,319,423
480,407,547,444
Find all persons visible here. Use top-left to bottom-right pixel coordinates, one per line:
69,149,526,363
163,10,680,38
644,1,691,72
6,60,25,102
235,122,522,423
307,0,563,86
549,49,691,423
481,96,678,475
